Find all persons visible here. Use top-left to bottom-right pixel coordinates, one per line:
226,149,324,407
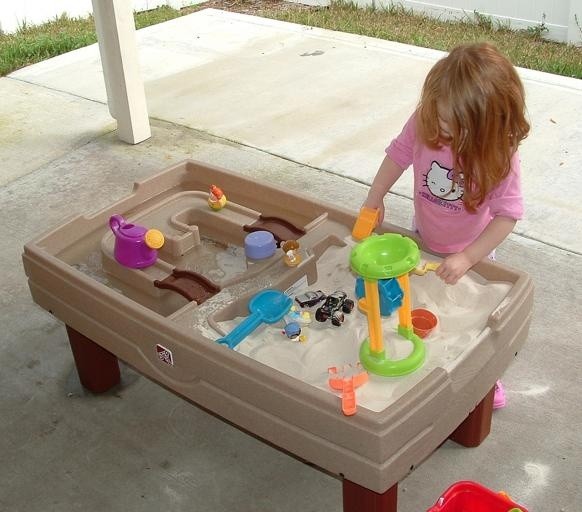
362,40,531,412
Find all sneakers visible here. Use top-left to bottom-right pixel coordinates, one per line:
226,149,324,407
492,380,505,408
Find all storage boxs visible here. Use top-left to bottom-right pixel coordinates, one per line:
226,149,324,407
428,481,528,512
